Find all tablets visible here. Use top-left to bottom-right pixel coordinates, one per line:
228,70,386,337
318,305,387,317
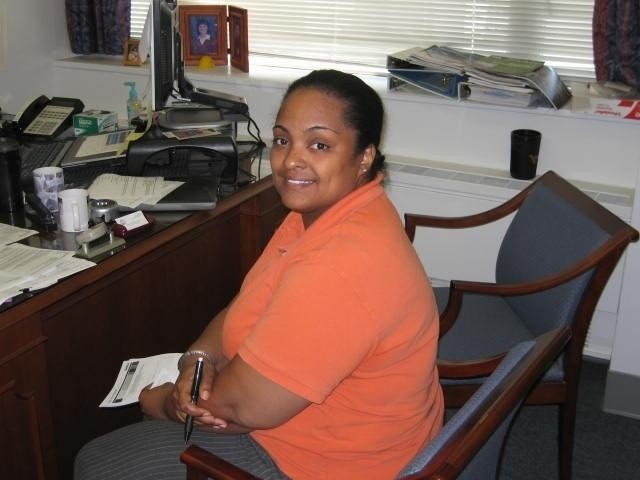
60,126,135,167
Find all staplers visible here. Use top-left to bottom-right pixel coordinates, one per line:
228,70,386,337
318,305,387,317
24,194,57,226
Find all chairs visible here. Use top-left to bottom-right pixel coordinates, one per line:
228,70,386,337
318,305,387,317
181,323,574,480
403,171,639,479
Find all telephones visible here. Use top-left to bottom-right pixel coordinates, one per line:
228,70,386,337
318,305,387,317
17,94,84,141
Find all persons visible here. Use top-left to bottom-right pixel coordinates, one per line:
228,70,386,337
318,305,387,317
190,18,217,53
70,69,447,480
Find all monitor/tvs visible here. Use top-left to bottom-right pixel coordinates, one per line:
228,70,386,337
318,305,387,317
150,1,249,131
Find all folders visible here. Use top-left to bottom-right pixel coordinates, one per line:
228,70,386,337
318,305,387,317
388,47,467,102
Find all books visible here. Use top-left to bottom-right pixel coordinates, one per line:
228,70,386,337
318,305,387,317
384,41,573,111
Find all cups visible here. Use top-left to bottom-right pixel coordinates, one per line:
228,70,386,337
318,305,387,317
0,137,24,213
34,166,90,234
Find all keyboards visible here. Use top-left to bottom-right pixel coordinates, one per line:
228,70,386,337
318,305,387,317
20,139,74,185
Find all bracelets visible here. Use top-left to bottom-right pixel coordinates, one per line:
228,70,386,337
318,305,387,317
177,349,214,372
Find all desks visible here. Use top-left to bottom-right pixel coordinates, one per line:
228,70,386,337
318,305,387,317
0,158,292,479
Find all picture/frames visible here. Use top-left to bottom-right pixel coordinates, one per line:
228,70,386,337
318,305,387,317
124,4,248,71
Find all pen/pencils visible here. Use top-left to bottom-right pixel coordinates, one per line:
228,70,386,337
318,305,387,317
184,356,204,445
